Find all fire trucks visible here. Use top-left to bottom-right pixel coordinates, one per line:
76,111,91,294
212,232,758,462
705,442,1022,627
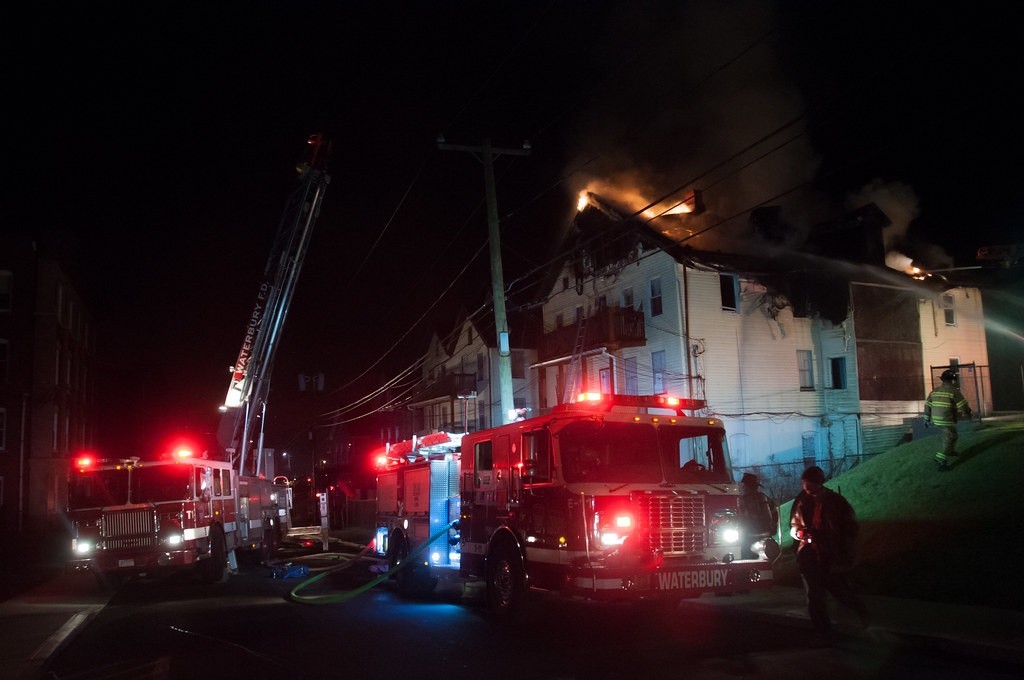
373,391,782,624
47,128,333,587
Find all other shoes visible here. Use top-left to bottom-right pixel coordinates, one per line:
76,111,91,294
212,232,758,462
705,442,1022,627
951,451,958,456
934,460,947,472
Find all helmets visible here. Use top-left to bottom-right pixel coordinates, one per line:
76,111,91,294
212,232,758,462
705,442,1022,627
939,370,956,380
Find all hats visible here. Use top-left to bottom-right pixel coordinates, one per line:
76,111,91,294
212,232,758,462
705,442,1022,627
801,467,824,486
740,473,758,489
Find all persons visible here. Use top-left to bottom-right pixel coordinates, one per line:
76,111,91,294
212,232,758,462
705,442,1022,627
741,472,779,536
789,466,860,646
922,370,973,471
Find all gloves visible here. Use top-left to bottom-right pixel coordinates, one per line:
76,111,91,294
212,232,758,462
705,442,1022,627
923,421,929,430
964,406,973,420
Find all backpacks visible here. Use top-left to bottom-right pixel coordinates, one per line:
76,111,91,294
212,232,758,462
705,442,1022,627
755,492,777,535
